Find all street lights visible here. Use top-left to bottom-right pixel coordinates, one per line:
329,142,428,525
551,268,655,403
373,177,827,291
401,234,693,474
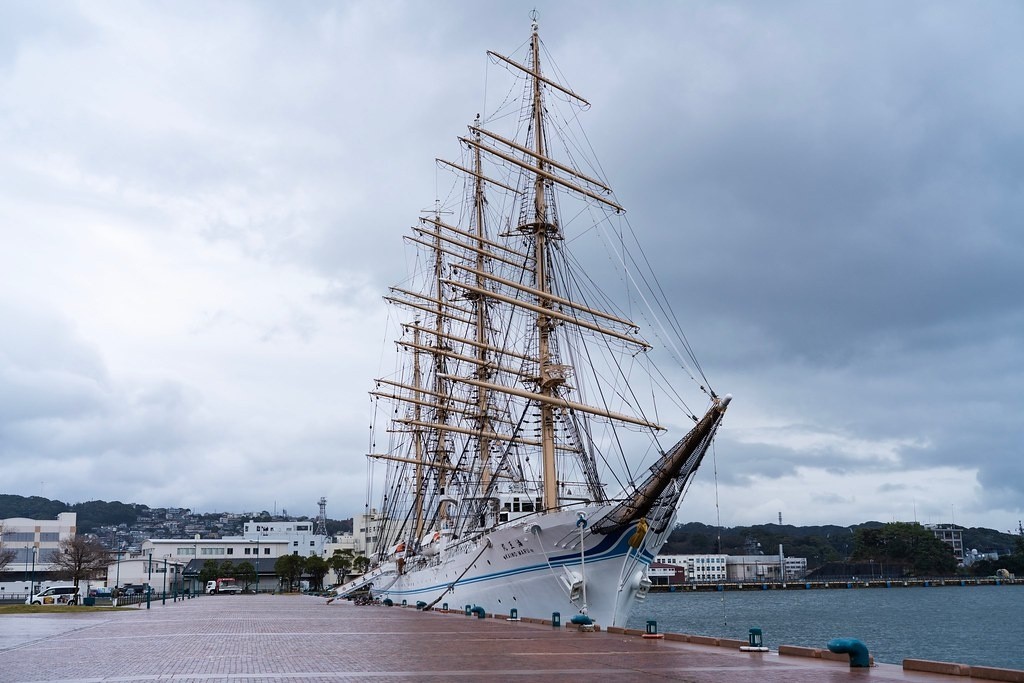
279,576,282,593
188,566,193,599
25,546,30,581
182,564,186,601
173,559,180,602
162,554,168,604
193,569,196,598
250,540,260,594
197,570,201,597
147,548,155,609
31,547,38,604
116,534,122,598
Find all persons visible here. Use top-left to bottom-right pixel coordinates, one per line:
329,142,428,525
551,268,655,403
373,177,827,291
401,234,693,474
112,586,119,608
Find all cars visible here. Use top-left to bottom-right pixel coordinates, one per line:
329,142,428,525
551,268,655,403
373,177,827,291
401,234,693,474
326,585,333,592
126,588,135,597
146,588,155,594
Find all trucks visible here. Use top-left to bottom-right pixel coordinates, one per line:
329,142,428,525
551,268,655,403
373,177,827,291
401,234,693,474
205,578,242,595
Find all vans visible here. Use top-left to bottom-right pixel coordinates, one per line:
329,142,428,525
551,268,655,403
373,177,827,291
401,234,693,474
25,585,84,607
122,585,144,596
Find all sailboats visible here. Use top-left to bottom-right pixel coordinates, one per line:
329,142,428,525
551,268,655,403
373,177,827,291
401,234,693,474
361,8,733,630
